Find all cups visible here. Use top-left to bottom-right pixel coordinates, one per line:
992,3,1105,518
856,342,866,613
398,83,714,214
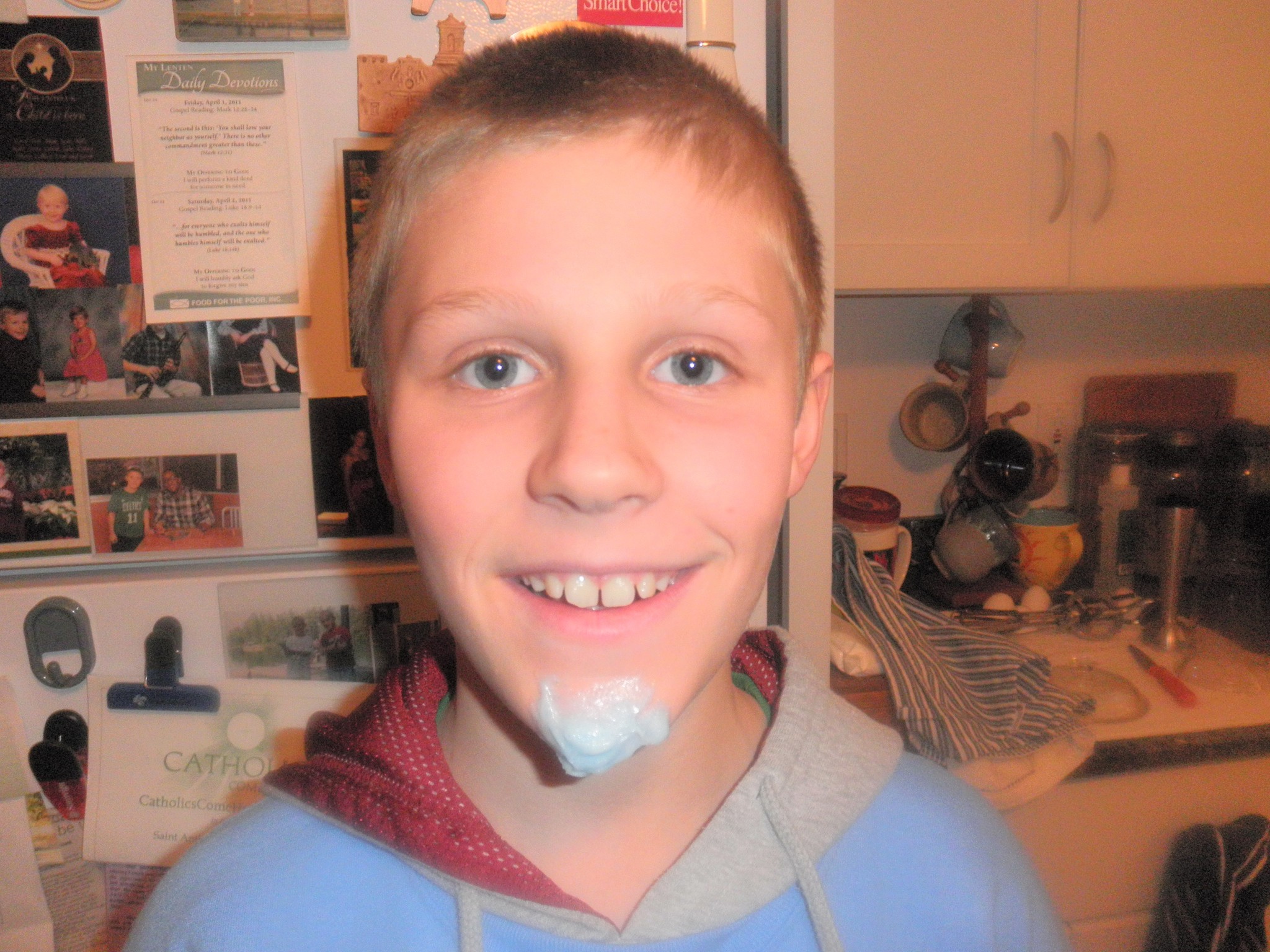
940,294,1025,378
931,493,1021,587
899,374,970,452
1007,509,1084,592
971,411,1059,502
853,526,912,591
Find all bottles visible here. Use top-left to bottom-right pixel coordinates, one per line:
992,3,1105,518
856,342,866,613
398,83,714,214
1151,495,1198,651
1203,425,1270,582
1135,428,1203,584
1074,428,1153,572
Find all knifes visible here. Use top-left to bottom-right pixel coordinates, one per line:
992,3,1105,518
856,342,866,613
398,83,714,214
1130,644,1197,708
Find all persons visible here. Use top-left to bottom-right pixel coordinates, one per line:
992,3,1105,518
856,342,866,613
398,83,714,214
0,301,46,402
153,468,215,535
24,184,109,288
107,466,150,552
121,323,202,398
63,305,107,399
341,428,375,536
218,317,298,392
286,610,355,682
121,24,1067,952
0,436,73,542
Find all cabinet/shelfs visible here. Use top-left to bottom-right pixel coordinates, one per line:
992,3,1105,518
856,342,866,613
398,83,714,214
833,0,1270,287
1002,753,1269,952
